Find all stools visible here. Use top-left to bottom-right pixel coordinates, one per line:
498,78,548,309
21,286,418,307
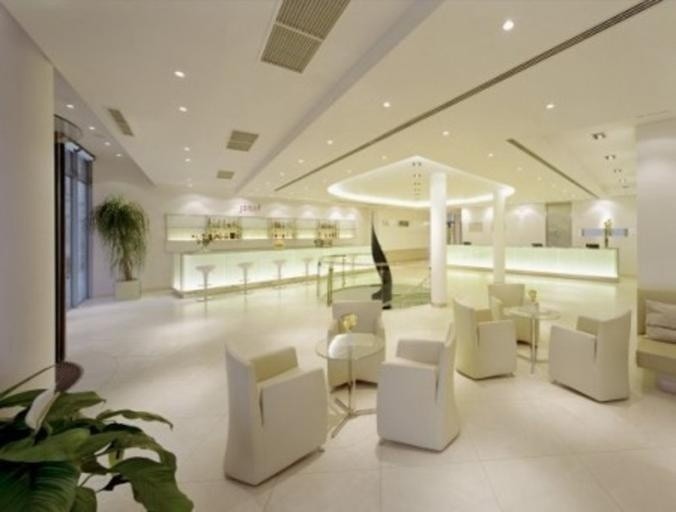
197,264,214,304
272,260,287,290
238,262,253,306
304,255,319,283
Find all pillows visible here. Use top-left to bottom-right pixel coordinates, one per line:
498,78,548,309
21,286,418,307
645,299,676,344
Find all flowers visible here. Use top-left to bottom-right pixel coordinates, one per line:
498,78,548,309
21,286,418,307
342,313,356,330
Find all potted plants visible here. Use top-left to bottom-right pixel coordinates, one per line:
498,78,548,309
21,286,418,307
84,198,151,302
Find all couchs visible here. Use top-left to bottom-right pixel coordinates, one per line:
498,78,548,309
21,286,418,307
634,288,676,390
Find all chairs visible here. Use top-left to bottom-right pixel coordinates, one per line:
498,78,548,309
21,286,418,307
486,281,537,349
453,297,520,377
224,343,328,487
378,318,461,454
547,310,633,403
325,299,388,388
